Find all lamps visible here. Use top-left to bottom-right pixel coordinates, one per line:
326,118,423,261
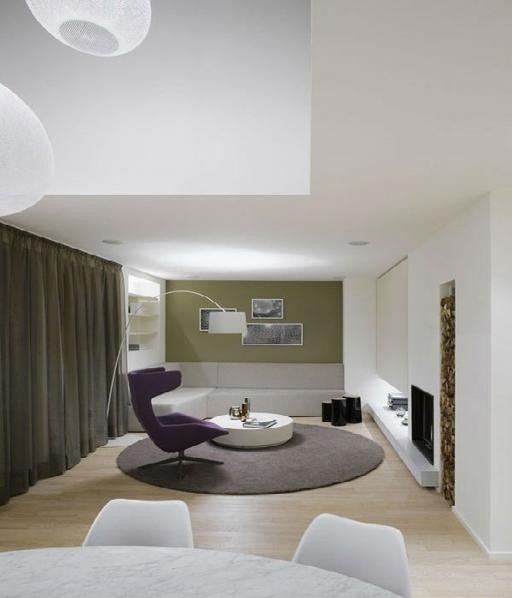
24,0,153,59
0,84,54,216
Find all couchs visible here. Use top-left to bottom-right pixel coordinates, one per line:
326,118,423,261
123,360,344,430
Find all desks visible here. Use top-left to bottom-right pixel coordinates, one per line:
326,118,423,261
0,547,406,598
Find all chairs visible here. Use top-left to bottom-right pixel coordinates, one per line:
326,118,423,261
292,513,414,598
128,367,230,484
83,499,196,547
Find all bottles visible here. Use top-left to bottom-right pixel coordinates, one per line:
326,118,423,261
240,396,250,422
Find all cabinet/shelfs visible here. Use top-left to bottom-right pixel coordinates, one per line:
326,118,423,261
127,290,158,350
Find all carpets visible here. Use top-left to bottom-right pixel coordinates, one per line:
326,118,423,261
116,421,386,495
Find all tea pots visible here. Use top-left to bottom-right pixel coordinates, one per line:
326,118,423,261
229,406,242,420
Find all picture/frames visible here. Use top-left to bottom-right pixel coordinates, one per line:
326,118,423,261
197,298,305,348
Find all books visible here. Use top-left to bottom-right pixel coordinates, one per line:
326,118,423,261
388,394,408,411
243,417,278,429
